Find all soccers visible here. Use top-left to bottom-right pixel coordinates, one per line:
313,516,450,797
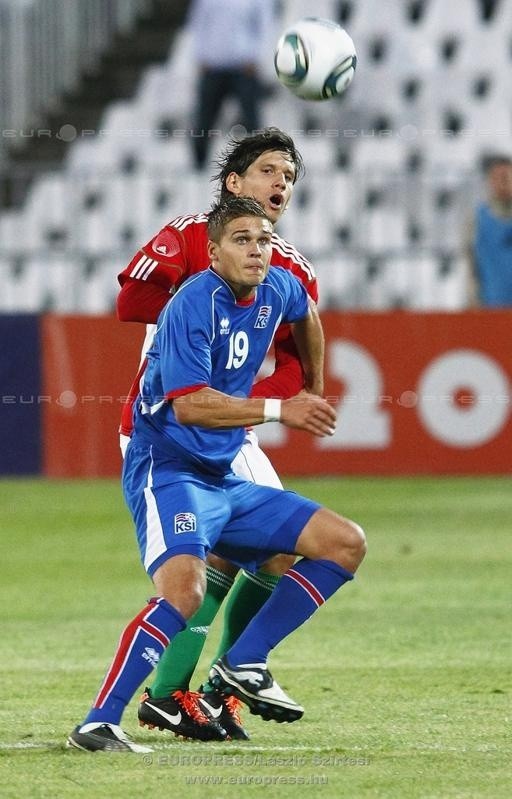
275,17,357,100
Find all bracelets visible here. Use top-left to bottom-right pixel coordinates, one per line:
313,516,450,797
261,395,284,424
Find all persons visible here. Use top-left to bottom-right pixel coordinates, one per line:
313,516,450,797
112,127,319,746
461,146,512,316
185,0,284,172
63,198,369,758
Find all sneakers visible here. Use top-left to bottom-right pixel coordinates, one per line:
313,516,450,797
136,687,229,741
208,653,305,724
193,680,251,740
65,722,156,754
